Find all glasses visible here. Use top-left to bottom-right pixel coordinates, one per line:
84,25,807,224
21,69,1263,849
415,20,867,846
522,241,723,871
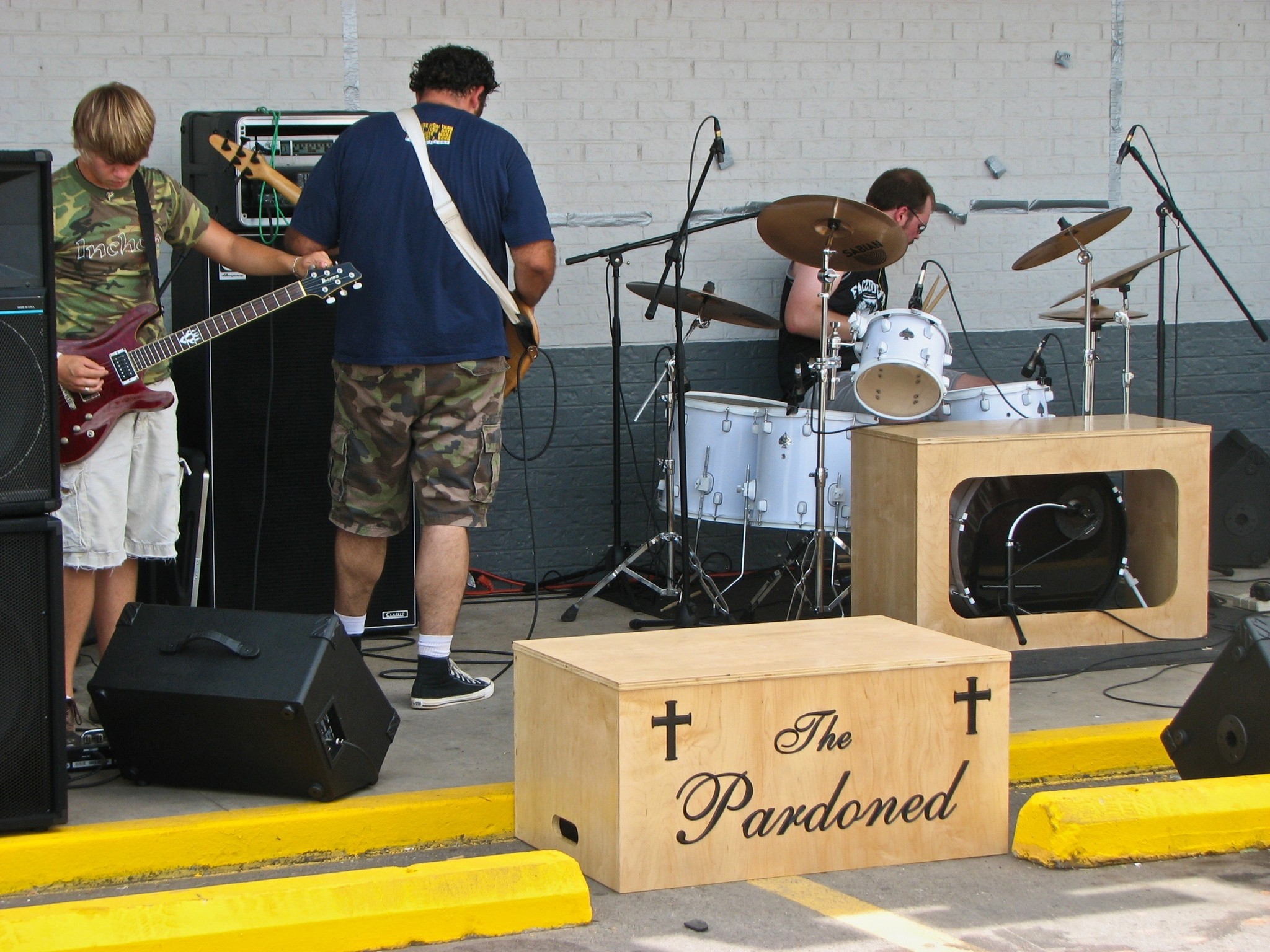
898,204,927,235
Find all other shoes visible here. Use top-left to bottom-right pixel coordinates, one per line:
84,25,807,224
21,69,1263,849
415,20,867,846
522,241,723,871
87,703,100,722
64,699,83,733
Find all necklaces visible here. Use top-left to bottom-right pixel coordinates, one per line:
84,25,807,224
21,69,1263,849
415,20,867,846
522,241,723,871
105,185,115,202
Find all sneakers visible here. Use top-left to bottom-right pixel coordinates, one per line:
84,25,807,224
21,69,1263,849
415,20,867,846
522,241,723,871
411,654,495,709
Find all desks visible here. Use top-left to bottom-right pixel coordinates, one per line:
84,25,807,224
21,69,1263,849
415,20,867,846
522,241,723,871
851,413,1212,651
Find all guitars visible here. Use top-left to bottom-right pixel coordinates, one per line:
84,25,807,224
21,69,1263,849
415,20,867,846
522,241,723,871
203,130,538,397
56,260,364,465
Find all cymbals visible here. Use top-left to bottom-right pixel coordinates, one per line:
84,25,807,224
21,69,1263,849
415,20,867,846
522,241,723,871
623,280,782,328
756,194,910,272
1050,242,1193,310
1037,304,1152,325
1010,205,1134,271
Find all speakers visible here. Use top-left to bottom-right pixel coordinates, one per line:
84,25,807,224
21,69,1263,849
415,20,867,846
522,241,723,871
0,148,68,832
170,233,426,635
1159,609,1270,780
1210,428,1270,568
87,601,400,801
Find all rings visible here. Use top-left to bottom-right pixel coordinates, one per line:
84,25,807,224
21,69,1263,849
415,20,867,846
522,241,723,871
85,386,90,395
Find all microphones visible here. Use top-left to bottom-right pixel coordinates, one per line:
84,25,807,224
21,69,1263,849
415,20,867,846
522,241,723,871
714,119,724,163
794,356,805,403
907,261,928,310
667,347,691,393
1021,334,1050,378
1116,126,1136,164
1067,498,1097,520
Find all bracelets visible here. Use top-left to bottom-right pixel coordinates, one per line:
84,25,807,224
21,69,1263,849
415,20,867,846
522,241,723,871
292,255,304,279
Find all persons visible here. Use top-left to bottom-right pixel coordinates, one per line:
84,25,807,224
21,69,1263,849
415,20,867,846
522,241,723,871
288,44,556,711
51,83,333,739
777,167,998,417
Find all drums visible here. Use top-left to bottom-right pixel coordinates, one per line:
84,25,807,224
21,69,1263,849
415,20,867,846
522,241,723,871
948,471,1127,618
746,407,878,534
938,379,1056,419
850,310,954,420
800,368,861,410
661,392,787,526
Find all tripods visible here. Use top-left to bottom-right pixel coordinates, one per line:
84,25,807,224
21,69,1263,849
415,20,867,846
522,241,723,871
524,139,850,631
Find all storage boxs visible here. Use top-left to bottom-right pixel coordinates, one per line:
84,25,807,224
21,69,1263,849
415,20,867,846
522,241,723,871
509,615,1012,893
